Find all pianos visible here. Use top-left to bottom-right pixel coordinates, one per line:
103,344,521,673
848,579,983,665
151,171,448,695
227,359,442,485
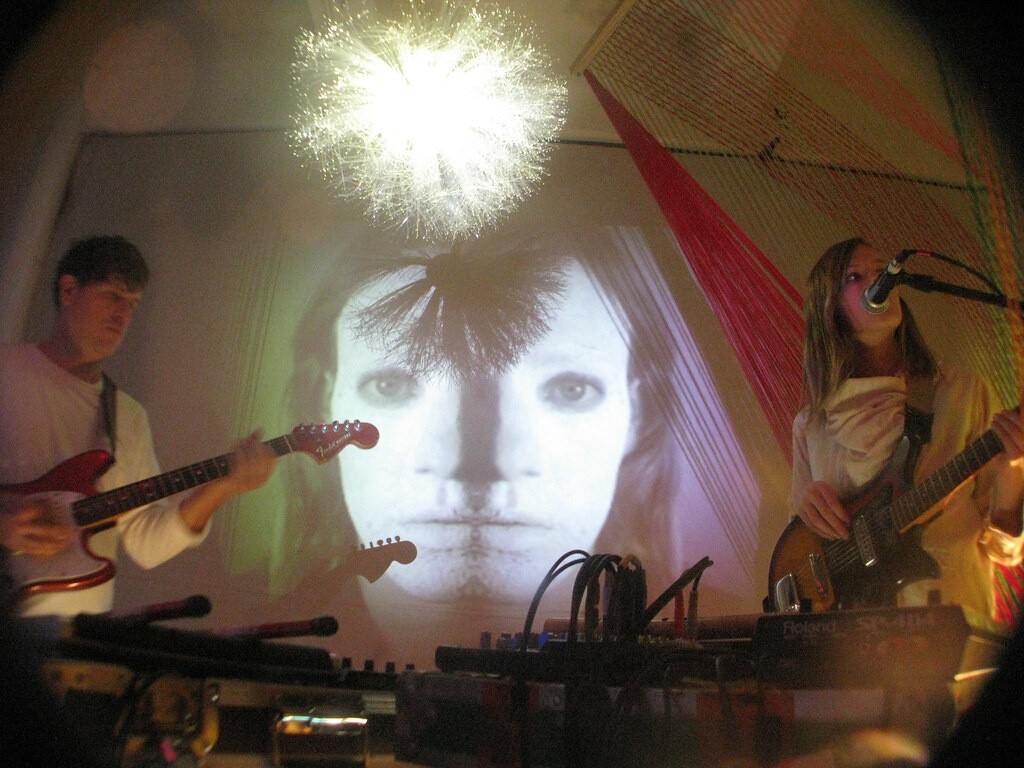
539,601,1018,685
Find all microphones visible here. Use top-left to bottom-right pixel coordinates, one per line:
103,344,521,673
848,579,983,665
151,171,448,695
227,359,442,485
859,249,912,315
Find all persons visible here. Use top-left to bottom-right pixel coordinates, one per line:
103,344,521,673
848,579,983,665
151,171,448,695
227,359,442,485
786,239,1024,617
291,220,683,659
0,234,279,614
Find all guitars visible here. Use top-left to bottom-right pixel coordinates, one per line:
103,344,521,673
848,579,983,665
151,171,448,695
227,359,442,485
767,400,1024,612
0,417,381,618
232,534,419,627
